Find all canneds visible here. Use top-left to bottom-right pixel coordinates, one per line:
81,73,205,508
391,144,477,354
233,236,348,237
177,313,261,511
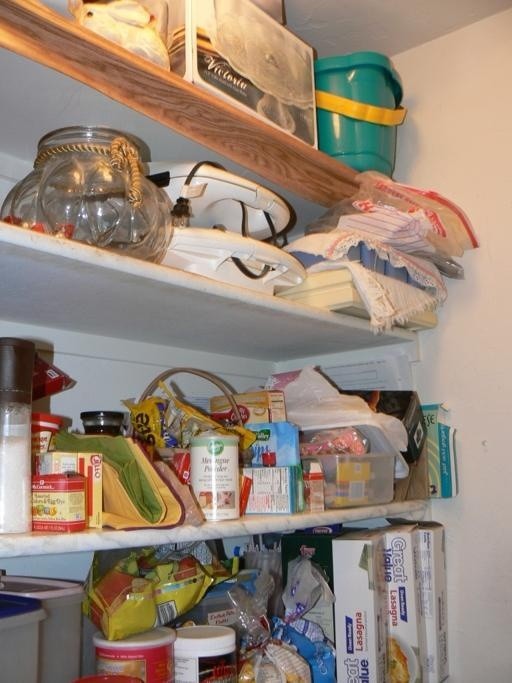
80,411,124,436
92,626,176,683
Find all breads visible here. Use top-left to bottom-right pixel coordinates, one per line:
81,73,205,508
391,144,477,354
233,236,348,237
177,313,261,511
238,658,304,683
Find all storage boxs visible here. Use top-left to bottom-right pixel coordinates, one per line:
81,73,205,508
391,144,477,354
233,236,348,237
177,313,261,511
303,453,394,509
1,571,87,681
163,0,318,151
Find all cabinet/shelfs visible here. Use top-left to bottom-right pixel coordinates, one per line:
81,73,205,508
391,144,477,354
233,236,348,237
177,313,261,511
1,0,431,557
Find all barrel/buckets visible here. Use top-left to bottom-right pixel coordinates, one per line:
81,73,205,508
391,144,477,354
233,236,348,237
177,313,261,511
313,51,405,178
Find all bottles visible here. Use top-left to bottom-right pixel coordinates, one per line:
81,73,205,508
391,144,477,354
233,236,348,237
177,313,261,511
93,625,237,681
0,126,174,265
0,336,123,537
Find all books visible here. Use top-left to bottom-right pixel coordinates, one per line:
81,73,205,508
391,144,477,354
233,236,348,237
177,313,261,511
272,268,438,333
